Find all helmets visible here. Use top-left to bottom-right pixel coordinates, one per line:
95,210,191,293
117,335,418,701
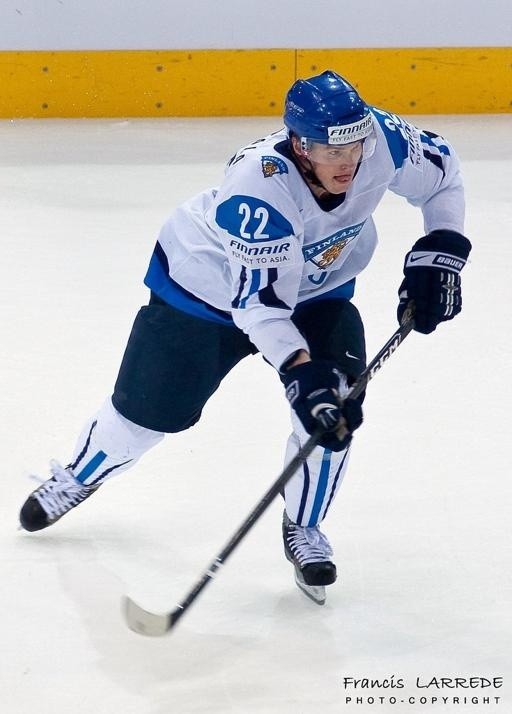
283,71,377,164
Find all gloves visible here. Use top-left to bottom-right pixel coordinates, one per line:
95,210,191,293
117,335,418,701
397,229,472,335
284,360,363,452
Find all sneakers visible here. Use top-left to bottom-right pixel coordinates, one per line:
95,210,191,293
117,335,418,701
20,459,104,532
282,509,337,586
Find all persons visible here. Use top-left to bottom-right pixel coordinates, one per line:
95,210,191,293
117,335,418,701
20,68,470,585
